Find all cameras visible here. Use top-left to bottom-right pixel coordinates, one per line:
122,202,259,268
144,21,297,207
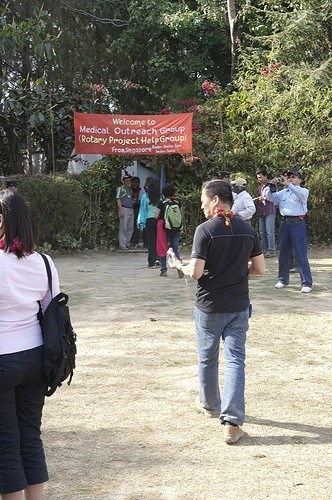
274,178,282,183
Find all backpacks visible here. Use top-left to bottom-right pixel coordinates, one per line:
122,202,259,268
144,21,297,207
33,249,78,397
161,199,183,231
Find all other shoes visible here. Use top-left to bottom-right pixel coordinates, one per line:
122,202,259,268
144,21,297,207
223,425,245,444
177,269,184,278
262,249,276,258
194,395,220,418
160,271,167,277
301,287,313,293
275,281,285,289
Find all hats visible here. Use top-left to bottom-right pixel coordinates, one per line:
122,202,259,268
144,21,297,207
231,178,247,188
287,170,301,178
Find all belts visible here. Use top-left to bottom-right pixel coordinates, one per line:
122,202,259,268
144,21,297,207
122,205,134,209
281,216,304,222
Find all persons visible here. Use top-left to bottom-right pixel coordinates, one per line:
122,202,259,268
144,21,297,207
116,174,166,250
262,170,313,293
0,189,61,500
157,186,184,278
167,180,266,443
137,179,165,268
256,168,277,258
228,177,256,227
